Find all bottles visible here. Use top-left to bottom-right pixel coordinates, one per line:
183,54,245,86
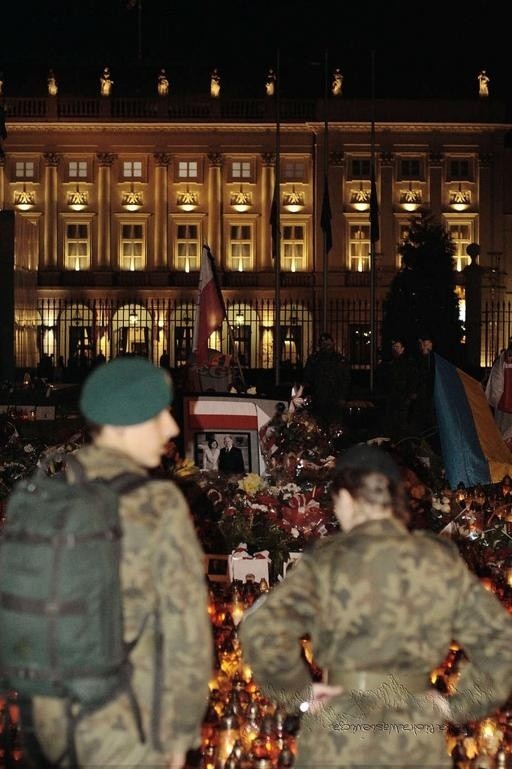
433,641,512,768
200,577,326,769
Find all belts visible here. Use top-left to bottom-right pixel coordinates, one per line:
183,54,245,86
325,672,432,693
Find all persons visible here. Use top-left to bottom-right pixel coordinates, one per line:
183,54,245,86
2,332,511,769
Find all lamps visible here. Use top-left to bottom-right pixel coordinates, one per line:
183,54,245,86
65,189,90,214
449,191,471,214
175,192,202,215
120,192,143,215
281,194,309,215
397,189,423,214
0,574,512,769
15,188,36,213
227,191,254,218
347,189,371,213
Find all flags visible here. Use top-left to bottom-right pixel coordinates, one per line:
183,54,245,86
369,136,381,244
269,128,281,257
320,126,333,252
191,246,228,364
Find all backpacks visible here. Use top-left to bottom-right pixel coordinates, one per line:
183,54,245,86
1,448,168,740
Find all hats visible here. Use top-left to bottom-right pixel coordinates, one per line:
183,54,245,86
77,356,174,429
330,445,395,472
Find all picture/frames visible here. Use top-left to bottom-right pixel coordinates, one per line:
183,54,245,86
194,432,252,473
